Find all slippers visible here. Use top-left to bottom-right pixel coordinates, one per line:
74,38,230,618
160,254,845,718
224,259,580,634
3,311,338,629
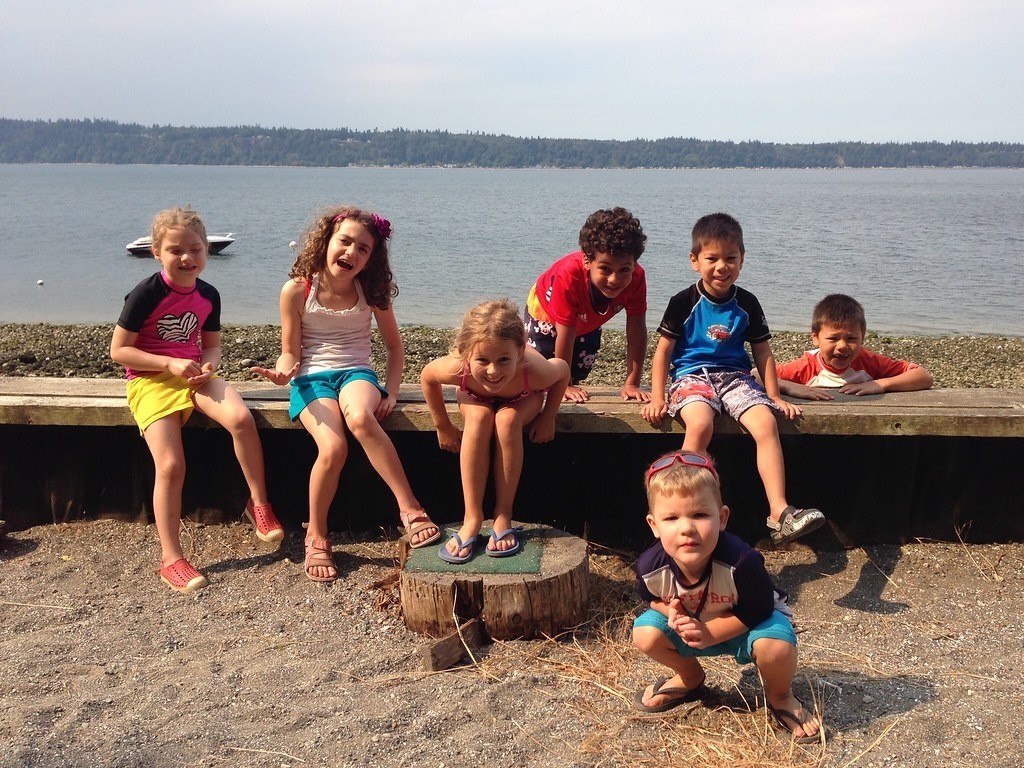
439,533,477,563
485,527,520,557
768,694,826,743
634,677,709,712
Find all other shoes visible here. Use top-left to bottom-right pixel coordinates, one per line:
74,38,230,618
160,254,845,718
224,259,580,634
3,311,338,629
160,558,208,593
244,498,284,542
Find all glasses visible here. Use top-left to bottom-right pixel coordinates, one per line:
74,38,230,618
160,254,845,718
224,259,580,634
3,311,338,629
646,453,720,489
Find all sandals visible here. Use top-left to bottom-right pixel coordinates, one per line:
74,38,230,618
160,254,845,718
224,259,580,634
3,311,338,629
766,506,826,545
304,537,338,582
400,507,440,549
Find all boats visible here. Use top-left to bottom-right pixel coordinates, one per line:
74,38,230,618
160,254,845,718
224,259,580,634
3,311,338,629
125,231,237,258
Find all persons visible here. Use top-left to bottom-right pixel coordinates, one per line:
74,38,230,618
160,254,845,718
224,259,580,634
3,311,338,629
750,294,933,401
642,213,824,544
421,301,570,564
110,209,284,592
523,206,652,403
252,206,438,583
632,451,824,742
